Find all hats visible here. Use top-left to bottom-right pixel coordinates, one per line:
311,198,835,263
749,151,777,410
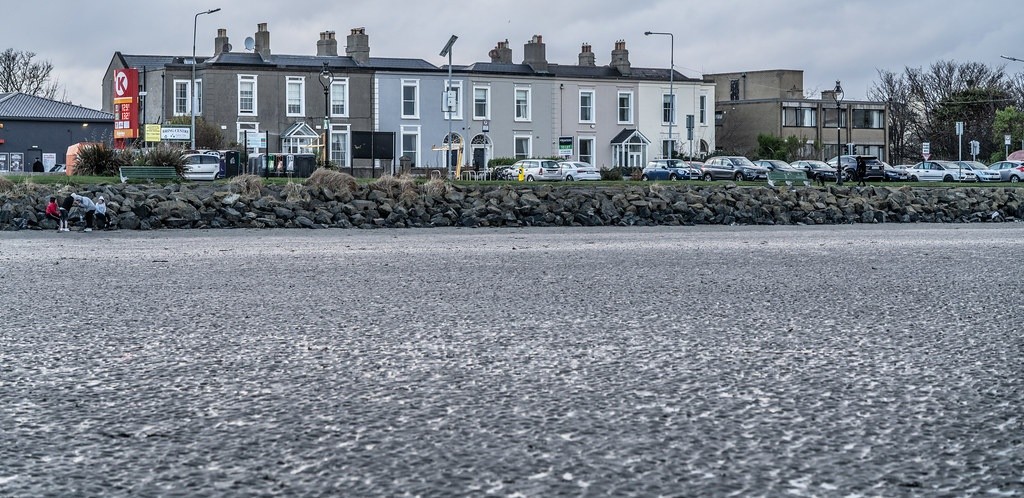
99,196,104,201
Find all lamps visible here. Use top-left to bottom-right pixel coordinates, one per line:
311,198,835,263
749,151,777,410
82,123,88,127
590,124,595,128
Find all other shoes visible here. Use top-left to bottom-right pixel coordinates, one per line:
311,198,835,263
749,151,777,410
84,228,92,232
64,229,70,231
60,228,64,231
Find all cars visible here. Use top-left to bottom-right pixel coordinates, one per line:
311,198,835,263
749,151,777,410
987,161,1024,183
503,159,565,182
641,159,702,182
956,161,1002,182
882,162,911,182
699,156,770,182
553,162,601,182
676,161,704,170
479,166,510,177
906,160,976,182
790,160,848,181
826,155,885,182
49,164,66,173
179,154,220,180
752,160,802,172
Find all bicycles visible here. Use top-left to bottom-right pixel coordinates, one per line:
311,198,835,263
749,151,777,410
497,170,515,181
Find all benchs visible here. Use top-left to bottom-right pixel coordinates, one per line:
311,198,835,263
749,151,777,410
119,165,182,184
765,171,810,188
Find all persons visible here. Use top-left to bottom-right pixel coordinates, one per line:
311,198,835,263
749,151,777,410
94,195,106,229
57,192,74,232
33,157,44,172
46,196,60,226
70,192,97,233
856,155,867,186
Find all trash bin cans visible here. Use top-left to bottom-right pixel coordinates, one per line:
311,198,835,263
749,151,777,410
225,150,240,178
261,152,316,178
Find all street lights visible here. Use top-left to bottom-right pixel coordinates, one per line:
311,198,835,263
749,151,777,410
192,8,221,150
644,32,674,159
318,62,334,169
833,81,844,186
